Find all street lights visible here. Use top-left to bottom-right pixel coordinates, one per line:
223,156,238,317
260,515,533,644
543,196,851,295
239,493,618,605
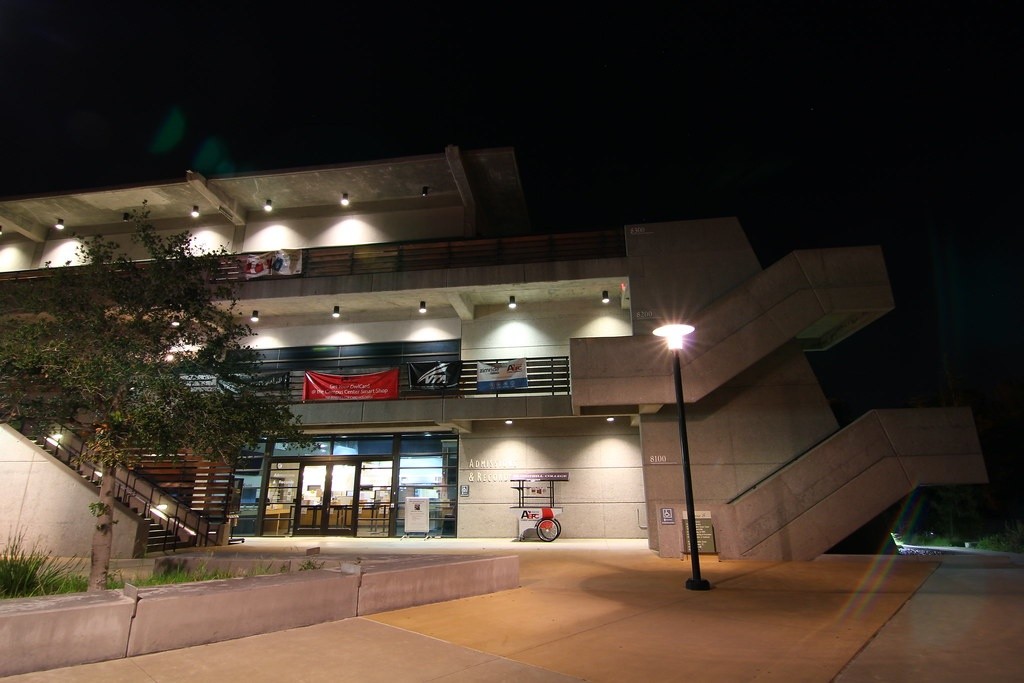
652,322,710,591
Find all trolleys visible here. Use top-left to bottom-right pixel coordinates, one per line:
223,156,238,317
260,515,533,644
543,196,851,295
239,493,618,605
510,473,569,542
